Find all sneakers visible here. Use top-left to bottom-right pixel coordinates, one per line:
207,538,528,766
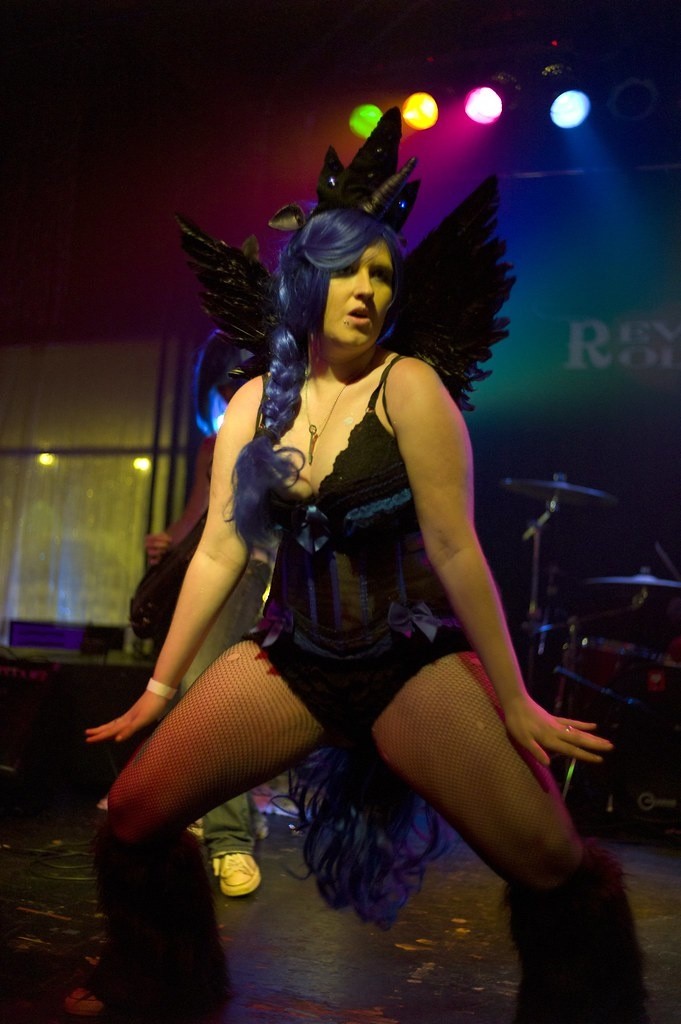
182,818,207,845
213,853,261,897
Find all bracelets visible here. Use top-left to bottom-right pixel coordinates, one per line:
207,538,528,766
146,677,178,700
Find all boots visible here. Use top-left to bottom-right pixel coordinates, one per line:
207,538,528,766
499,845,655,1024
64,811,239,1017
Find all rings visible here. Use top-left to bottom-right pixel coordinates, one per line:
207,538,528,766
113,719,118,725
567,724,574,736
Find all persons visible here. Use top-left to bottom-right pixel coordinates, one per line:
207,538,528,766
80,215,660,1024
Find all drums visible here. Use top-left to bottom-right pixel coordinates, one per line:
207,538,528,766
557,632,681,736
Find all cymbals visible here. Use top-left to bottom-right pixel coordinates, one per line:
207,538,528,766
497,477,622,508
582,571,681,593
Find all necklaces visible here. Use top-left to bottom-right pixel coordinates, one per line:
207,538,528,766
302,366,350,465
